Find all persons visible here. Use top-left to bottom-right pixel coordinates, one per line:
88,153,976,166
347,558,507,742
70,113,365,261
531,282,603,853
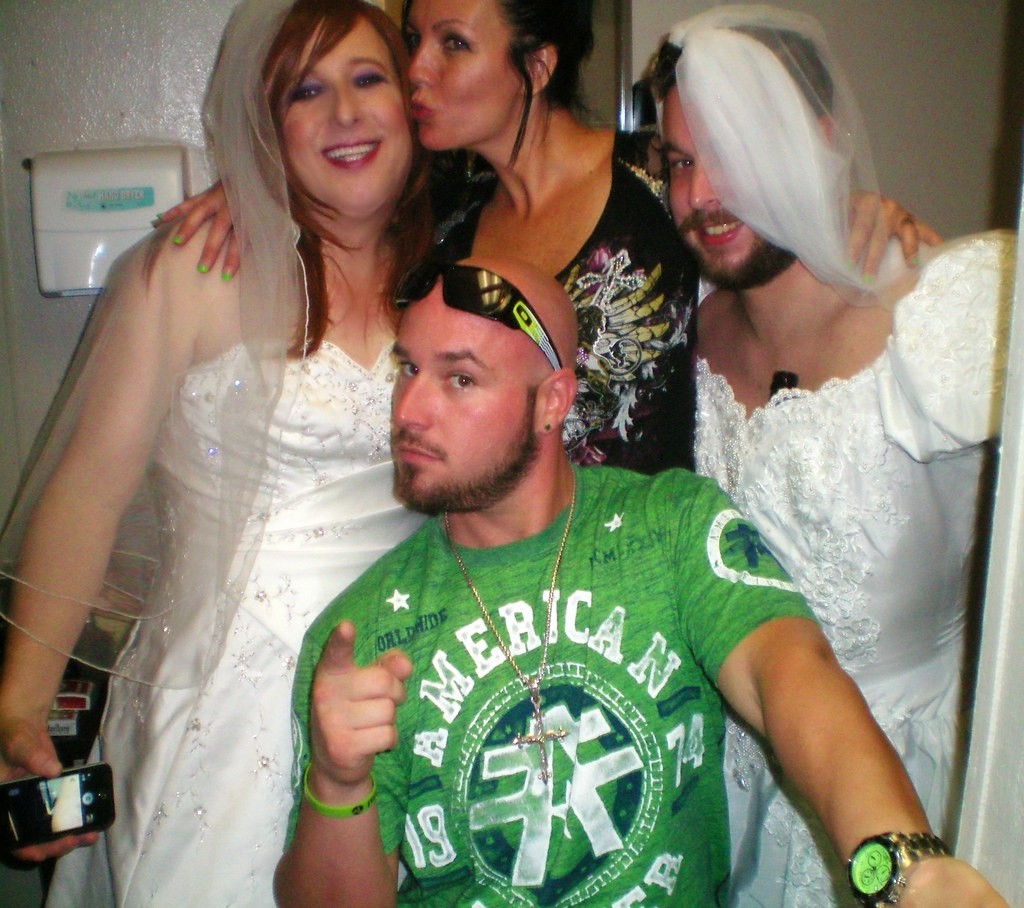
271,255,1023,908
156,0,951,477
0,0,434,908
651,2,1023,908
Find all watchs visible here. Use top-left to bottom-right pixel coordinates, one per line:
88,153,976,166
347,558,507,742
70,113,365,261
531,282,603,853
845,832,956,908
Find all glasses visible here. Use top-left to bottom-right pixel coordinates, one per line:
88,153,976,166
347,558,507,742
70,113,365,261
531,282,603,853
395,256,565,373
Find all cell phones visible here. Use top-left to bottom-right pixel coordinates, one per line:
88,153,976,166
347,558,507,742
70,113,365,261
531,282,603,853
0,763,116,849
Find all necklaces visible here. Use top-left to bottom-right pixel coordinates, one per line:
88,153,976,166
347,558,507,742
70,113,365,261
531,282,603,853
444,465,577,786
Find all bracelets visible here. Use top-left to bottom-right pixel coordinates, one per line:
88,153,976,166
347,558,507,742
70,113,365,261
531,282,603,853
303,762,379,819
